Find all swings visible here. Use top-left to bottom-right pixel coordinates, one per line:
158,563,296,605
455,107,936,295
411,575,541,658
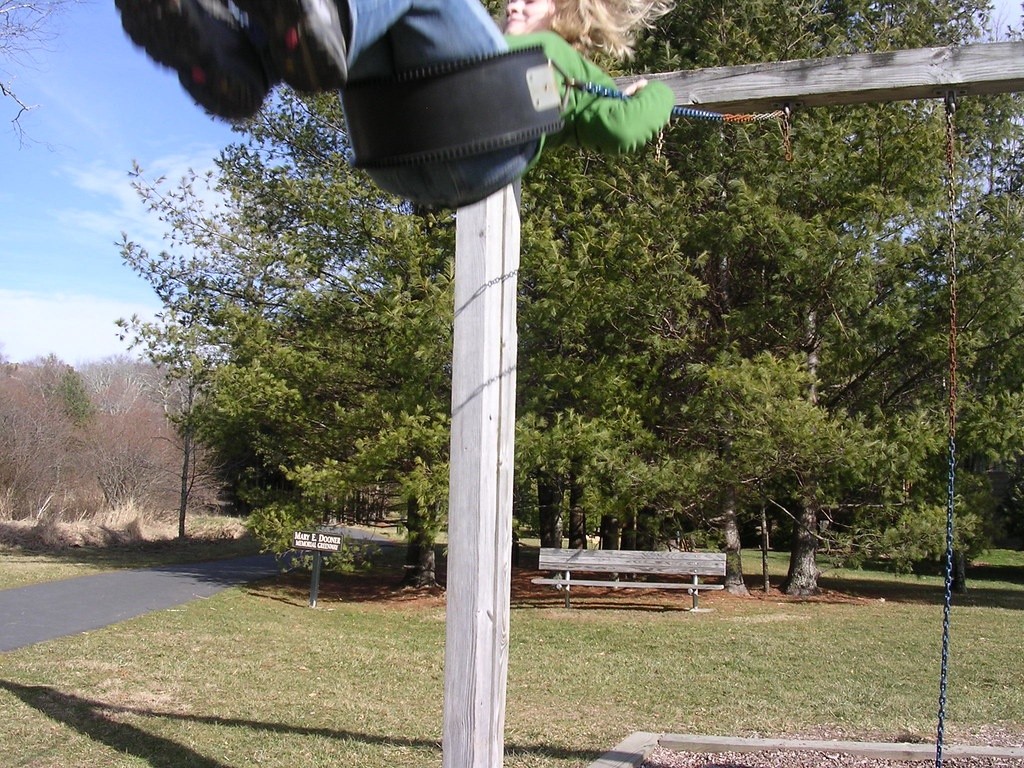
338,41,795,175
930,90,972,766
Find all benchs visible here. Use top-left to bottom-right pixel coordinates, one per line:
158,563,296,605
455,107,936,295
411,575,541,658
531,548,727,610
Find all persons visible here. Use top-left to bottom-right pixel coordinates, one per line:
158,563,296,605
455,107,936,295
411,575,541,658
114,1,675,211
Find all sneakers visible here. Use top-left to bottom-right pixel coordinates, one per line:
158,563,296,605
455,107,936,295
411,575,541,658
113,0,349,121
